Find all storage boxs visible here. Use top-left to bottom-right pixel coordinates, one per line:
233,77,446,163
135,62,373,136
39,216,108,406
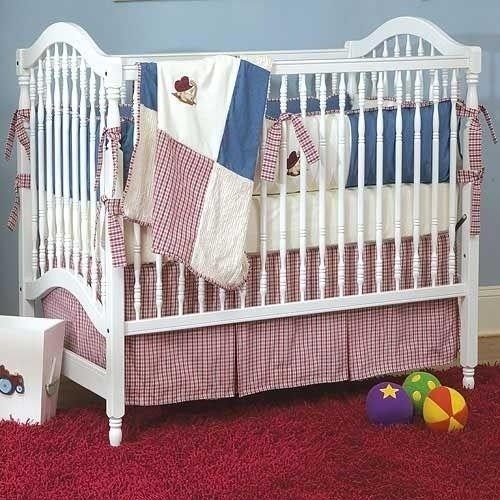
0,315,64,426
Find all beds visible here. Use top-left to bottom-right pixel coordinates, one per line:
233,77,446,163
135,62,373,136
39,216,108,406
16,17,482,447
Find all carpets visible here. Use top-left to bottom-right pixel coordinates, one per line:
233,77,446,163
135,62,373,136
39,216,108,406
0,363,500,500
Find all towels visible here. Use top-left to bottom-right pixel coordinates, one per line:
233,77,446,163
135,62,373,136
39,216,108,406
121,56,273,292
